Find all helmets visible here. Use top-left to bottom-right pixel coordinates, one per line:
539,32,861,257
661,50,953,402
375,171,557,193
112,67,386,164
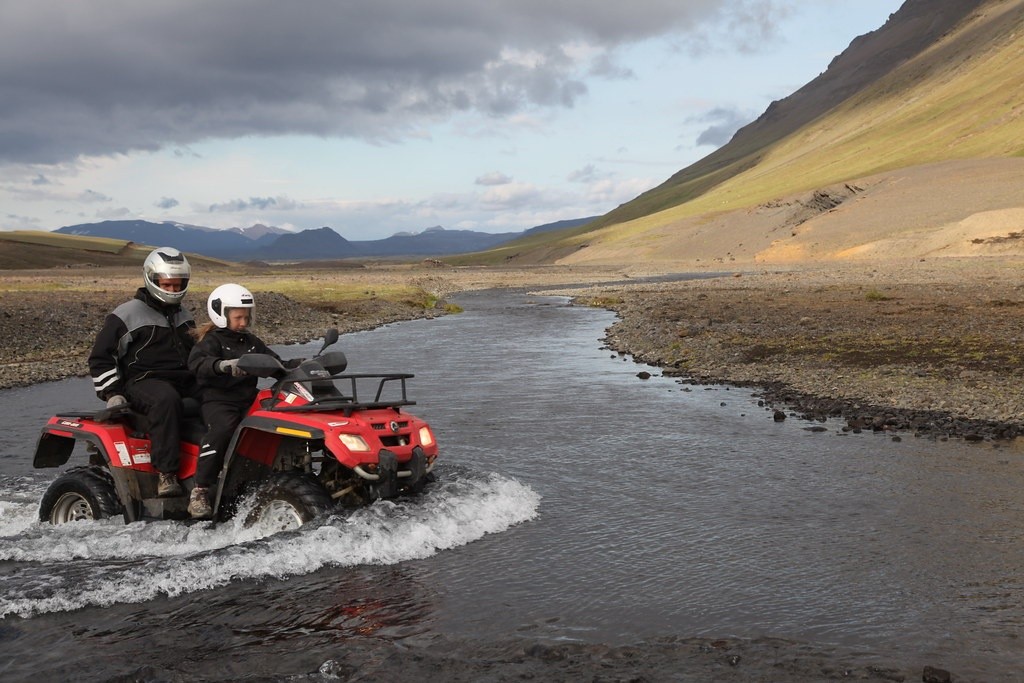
143,247,191,304
207,283,256,328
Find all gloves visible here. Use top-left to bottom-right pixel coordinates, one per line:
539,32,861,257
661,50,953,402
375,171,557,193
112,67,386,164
219,358,247,377
107,395,127,408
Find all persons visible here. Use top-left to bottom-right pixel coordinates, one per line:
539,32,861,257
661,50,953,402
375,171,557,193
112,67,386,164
88,247,198,495
188,284,306,519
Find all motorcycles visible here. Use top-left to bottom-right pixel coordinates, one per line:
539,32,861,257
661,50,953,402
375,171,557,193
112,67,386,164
31,325,444,545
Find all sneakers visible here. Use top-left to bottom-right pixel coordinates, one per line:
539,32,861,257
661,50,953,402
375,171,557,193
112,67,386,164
187,486,211,518
158,472,182,497
